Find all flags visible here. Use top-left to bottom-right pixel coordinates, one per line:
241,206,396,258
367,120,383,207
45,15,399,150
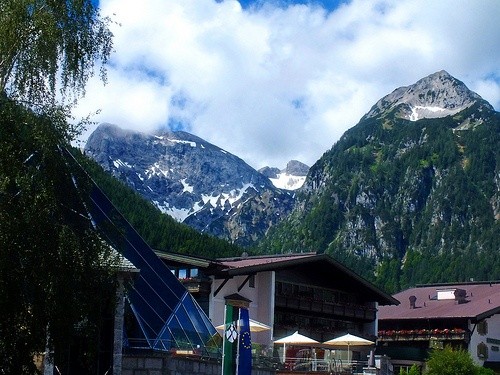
223,304,236,375
237,306,252,375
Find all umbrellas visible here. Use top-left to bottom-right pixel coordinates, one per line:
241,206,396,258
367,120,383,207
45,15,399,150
322,333,375,365
273,331,320,345
214,318,271,332
